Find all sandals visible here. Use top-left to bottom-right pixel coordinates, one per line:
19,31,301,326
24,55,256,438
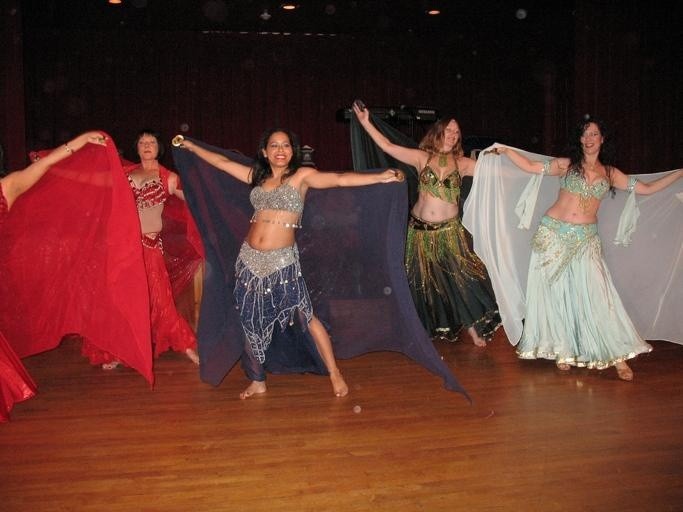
616,365,634,381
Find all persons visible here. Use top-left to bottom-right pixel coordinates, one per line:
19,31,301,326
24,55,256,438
0,131,107,425
33,128,200,371
351,101,535,348
176,125,405,400
492,114,681,380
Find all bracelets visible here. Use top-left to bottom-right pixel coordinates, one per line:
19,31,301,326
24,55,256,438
65,140,74,154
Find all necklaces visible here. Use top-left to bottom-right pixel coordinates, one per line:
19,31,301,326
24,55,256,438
585,163,600,170
